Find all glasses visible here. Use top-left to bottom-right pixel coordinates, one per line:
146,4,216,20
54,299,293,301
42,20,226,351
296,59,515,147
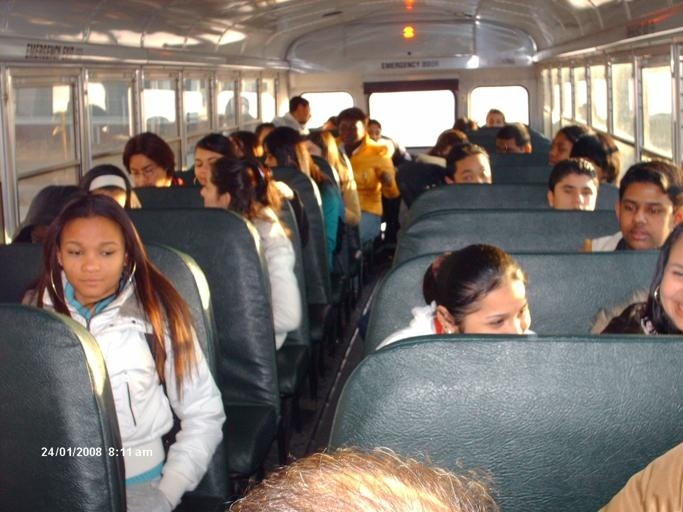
129,164,162,179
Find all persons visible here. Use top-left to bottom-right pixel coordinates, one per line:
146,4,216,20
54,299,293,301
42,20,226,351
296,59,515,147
263,127,342,278
22,195,227,512
228,445,499,511
598,443,683,512
568,132,620,184
496,121,532,154
11,184,82,244
376,244,537,351
322,116,339,132
432,128,468,157
337,107,400,258
305,131,361,226
123,133,191,188
486,109,506,128
256,123,274,144
546,158,598,211
201,156,302,352
194,134,240,185
588,220,683,334
581,160,683,252
453,118,479,132
548,125,591,165
271,96,312,136
229,131,263,156
81,164,143,209
367,120,412,167
445,144,492,184
